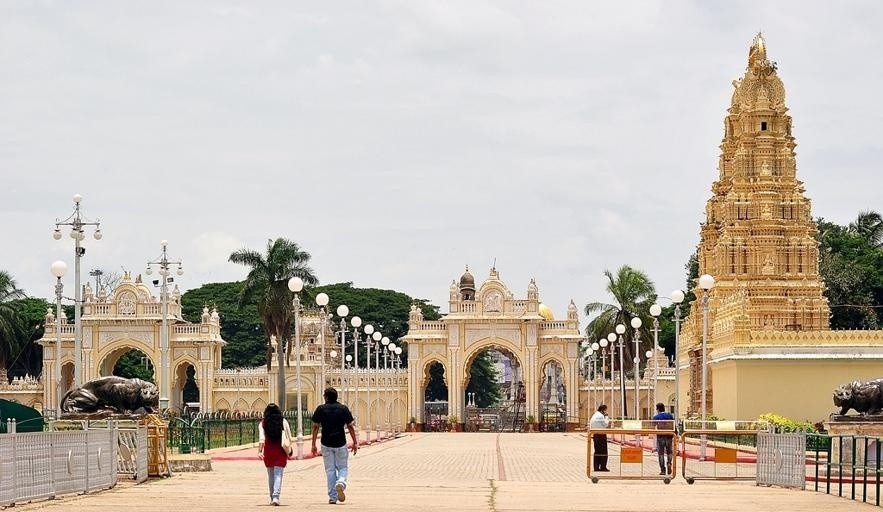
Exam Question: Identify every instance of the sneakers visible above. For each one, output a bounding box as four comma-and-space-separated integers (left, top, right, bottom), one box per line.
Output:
329, 483, 345, 504
271, 498, 279, 505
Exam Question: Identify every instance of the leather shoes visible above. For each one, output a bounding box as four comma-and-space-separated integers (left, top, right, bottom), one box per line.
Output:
594, 468, 609, 471
660, 464, 671, 475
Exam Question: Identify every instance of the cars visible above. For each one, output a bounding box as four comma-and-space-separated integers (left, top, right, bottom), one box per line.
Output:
471, 413, 503, 433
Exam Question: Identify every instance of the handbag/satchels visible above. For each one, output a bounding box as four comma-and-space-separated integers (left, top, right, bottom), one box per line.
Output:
281, 431, 292, 455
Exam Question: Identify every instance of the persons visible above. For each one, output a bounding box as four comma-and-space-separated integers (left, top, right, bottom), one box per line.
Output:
651, 402, 678, 475
589, 405, 611, 471
258, 402, 294, 506
310, 387, 358, 503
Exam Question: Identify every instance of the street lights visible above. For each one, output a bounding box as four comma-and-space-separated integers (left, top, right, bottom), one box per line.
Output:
146, 239, 186, 415
288, 276, 403, 461
577, 273, 715, 445
49, 190, 103, 425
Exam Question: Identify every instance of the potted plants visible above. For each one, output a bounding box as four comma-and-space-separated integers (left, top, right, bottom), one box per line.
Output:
410, 416, 416, 428
449, 414, 458, 429
528, 415, 535, 433
